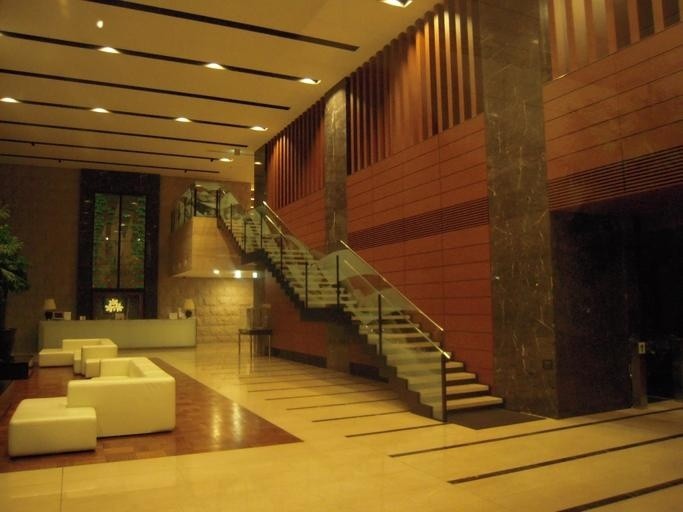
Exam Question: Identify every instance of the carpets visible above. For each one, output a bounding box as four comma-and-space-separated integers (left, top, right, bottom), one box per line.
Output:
0, 356, 304, 475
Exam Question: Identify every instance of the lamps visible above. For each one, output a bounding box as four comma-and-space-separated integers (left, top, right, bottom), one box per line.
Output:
183, 298, 195, 318
43, 298, 57, 320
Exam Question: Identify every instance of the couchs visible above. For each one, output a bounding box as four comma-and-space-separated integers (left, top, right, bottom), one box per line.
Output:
38, 338, 118, 376
8, 356, 176, 457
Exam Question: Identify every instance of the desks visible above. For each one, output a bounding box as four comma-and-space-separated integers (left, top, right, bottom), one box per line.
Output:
238, 328, 273, 362
